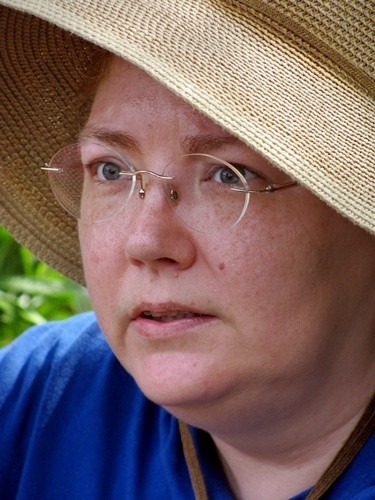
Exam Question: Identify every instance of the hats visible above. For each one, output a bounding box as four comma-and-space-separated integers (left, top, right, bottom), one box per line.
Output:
0, 0, 375, 284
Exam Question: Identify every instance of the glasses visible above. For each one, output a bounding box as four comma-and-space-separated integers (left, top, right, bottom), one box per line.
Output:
40, 139, 297, 236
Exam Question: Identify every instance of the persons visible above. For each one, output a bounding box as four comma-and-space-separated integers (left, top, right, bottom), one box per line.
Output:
0, 0, 375, 500
